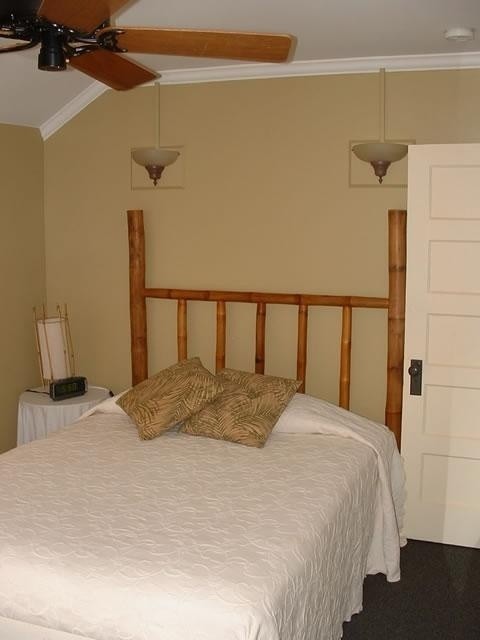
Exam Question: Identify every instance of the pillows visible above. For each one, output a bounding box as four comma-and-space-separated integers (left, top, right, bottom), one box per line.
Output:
114, 355, 306, 450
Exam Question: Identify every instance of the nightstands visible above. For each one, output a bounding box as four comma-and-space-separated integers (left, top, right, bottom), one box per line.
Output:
18, 384, 110, 445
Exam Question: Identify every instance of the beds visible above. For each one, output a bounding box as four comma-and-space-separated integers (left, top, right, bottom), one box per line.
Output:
0, 207, 409, 640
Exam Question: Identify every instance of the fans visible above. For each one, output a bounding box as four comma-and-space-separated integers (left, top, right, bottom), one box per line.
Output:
1, 0, 300, 94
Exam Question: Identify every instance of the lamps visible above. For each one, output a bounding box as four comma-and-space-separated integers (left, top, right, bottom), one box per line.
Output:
129, 81, 181, 188
31, 302, 76, 390
351, 66, 409, 186
